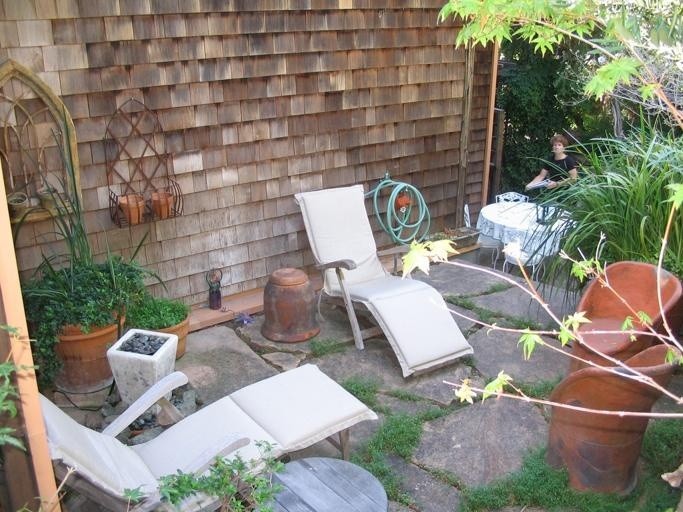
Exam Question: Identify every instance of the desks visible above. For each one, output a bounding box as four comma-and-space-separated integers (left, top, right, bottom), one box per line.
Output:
478, 200, 573, 280
253, 453, 392, 511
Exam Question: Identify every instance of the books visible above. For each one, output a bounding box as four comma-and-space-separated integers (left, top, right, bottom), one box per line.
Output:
526, 179, 550, 190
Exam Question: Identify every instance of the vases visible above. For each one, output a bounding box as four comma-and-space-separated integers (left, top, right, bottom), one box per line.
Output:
121, 190, 176, 223
105, 329, 178, 415
7, 186, 58, 215
259, 266, 320, 341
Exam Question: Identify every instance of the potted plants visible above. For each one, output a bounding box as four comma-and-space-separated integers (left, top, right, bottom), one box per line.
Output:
12, 103, 173, 393
128, 293, 192, 360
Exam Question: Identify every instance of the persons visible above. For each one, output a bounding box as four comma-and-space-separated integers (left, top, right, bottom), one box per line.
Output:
525, 137, 577, 203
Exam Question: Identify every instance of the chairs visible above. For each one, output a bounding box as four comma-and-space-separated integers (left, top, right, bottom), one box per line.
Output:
291, 184, 479, 379
24, 361, 378, 511
549, 258, 682, 498
495, 191, 530, 203
501, 229, 546, 281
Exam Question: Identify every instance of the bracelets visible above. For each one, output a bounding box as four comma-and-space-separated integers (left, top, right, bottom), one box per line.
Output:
555, 182, 560, 188
